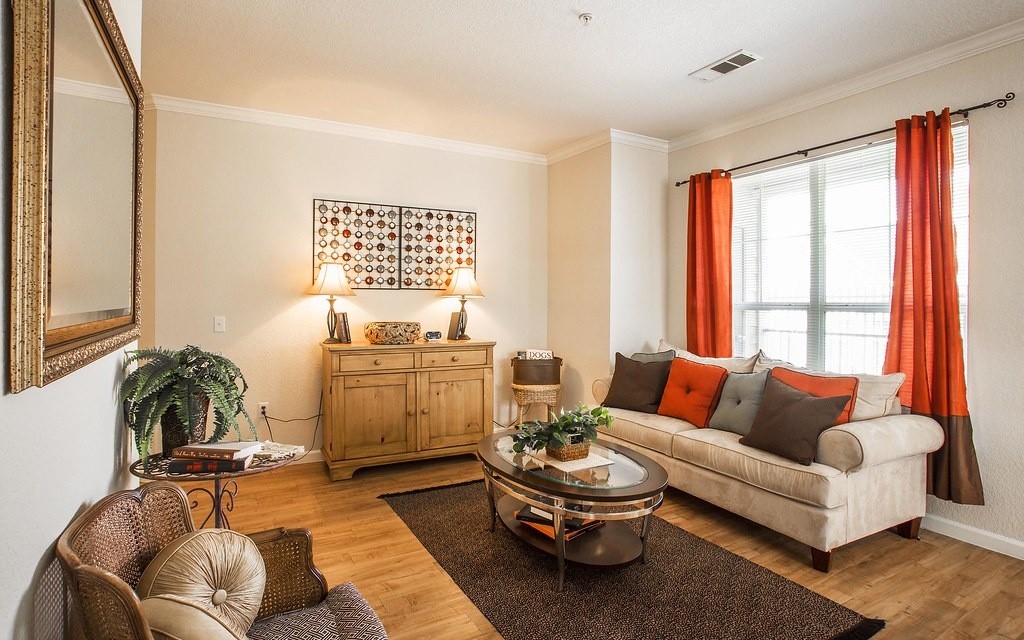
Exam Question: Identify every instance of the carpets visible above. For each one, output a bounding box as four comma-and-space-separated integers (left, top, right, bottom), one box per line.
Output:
377, 464, 887, 640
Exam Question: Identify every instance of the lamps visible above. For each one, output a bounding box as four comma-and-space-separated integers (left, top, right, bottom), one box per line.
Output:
308, 263, 360, 346
441, 266, 485, 340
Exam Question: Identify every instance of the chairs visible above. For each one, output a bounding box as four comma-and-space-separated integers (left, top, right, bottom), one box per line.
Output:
54, 478, 388, 640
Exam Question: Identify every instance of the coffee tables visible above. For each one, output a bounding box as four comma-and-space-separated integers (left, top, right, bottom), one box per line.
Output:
477, 427, 668, 592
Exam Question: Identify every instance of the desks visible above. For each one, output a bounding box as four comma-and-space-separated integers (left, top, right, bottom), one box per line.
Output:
130, 452, 296, 531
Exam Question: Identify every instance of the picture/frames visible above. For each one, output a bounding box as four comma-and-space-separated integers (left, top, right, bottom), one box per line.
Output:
10, 0, 146, 393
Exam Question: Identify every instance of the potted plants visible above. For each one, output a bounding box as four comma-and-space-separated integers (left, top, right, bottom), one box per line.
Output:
121, 343, 260, 475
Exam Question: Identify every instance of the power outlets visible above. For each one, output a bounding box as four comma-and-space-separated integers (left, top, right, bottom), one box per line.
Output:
259, 402, 268, 417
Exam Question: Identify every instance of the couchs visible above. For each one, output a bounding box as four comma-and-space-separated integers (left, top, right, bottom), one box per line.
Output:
592, 377, 945, 571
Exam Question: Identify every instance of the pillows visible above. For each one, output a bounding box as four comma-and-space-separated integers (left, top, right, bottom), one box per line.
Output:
137, 527, 265, 640
600, 338, 905, 466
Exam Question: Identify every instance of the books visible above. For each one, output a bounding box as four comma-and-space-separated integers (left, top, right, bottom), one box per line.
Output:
171, 440, 262, 461
513, 494, 606, 541
167, 454, 254, 472
335, 312, 352, 344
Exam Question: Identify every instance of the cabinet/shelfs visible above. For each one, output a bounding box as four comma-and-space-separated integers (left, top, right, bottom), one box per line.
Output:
318, 340, 497, 482
